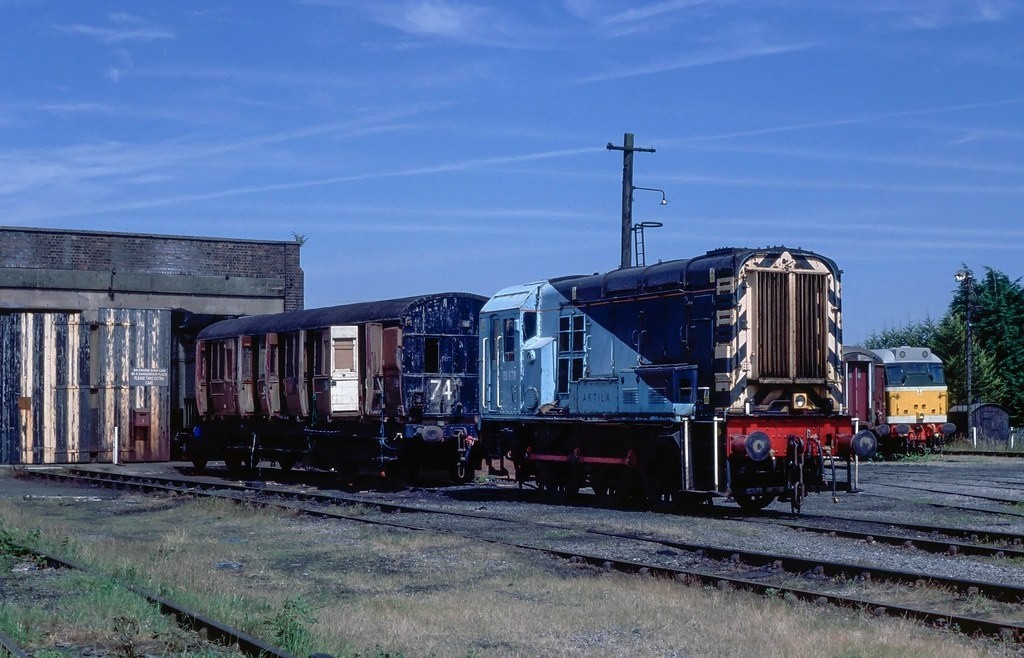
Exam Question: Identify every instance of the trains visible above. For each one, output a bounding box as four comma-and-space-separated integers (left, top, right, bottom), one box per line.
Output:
843, 346, 955, 455
175, 245, 852, 513
